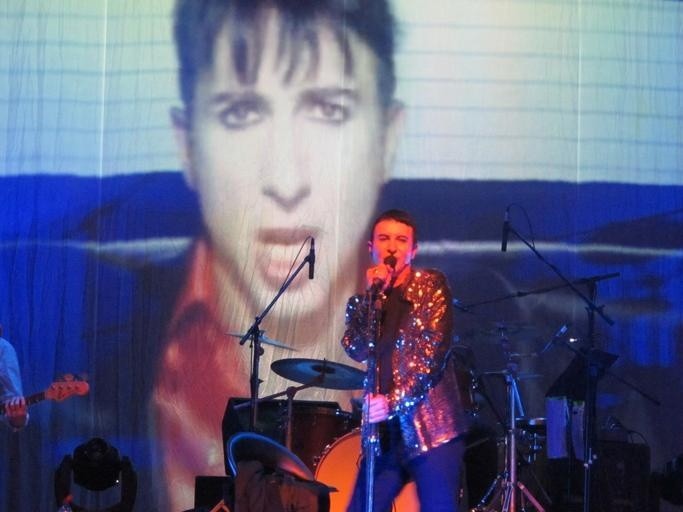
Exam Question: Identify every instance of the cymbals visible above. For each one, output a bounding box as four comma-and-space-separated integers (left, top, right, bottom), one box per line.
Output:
272, 358, 366, 390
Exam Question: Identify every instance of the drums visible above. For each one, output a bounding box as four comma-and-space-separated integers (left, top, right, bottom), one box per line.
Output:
454, 364, 479, 415
301, 405, 349, 463
316, 426, 467, 511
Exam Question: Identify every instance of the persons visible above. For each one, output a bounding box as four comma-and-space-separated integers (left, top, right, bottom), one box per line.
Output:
143, 1, 406, 512
341, 209, 472, 511
1, 338, 29, 511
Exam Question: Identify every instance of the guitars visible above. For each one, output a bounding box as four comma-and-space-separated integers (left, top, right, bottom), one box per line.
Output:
1, 376, 89, 414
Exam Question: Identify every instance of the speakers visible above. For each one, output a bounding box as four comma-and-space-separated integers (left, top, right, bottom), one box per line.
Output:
584, 442, 651, 512
221, 397, 351, 476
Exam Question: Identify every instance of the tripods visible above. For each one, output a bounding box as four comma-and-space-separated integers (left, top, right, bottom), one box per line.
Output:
470, 335, 553, 512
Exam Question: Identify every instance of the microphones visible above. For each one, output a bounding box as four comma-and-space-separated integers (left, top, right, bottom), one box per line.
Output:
543, 320, 569, 352
502, 208, 509, 251
452, 298, 469, 311
373, 256, 396, 289
309, 239, 315, 279
